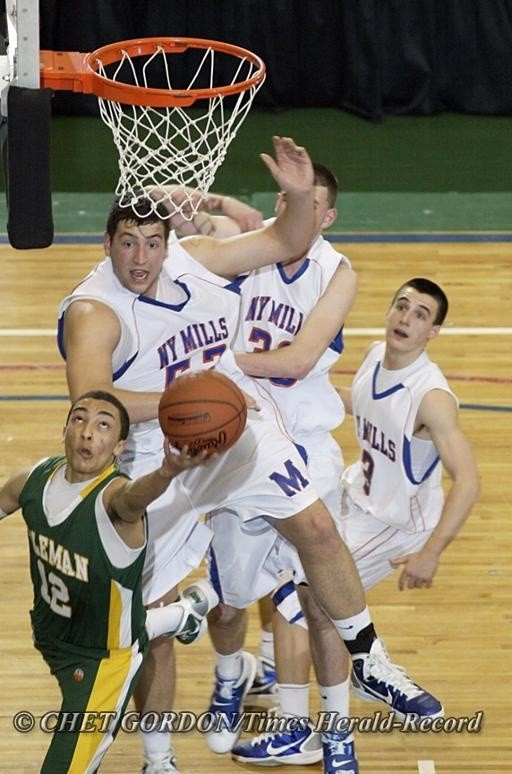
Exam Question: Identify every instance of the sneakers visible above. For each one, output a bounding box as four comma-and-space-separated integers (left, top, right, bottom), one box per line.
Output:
141, 638, 445, 773
166, 580, 220, 645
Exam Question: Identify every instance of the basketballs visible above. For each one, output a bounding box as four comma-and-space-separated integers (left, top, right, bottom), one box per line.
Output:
159, 370, 247, 459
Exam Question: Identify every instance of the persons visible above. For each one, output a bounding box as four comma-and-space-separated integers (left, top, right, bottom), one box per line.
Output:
0, 137, 479, 772
